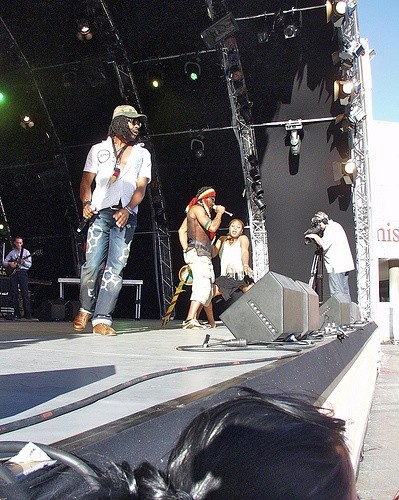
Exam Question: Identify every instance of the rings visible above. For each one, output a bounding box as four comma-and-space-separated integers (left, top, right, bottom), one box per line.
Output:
119, 212, 123, 215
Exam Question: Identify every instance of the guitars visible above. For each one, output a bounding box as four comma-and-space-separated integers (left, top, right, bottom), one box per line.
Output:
5, 249, 43, 277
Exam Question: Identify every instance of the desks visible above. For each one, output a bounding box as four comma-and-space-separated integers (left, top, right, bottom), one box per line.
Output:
57, 277, 144, 320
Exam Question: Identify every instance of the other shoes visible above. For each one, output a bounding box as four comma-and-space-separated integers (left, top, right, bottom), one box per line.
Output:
182, 319, 206, 330
25, 313, 30, 318
15, 312, 21, 318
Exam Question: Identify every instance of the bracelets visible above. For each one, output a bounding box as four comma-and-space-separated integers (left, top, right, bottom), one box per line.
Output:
244, 264, 249, 267
124, 206, 136, 216
82, 201, 91, 208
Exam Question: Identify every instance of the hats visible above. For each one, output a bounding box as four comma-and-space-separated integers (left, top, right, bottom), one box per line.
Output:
113, 105, 147, 122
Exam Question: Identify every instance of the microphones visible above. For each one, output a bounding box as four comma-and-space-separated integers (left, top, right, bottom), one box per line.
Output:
76, 204, 96, 232
212, 205, 233, 217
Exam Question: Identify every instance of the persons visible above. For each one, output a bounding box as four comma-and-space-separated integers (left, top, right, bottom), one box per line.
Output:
304, 211, 356, 331
76, 385, 361, 500
73, 105, 155, 333
2, 236, 31, 320
178, 185, 224, 329
211, 219, 255, 304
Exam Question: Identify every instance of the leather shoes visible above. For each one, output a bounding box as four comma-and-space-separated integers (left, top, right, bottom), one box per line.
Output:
73, 311, 91, 331
93, 323, 117, 336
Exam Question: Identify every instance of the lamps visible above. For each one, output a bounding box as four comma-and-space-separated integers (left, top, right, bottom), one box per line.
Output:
248, 155, 266, 210
339, 80, 353, 99
330, 1, 347, 23
75, 15, 92, 34
147, 64, 164, 89
285, 123, 303, 155
231, 63, 253, 125
281, 12, 299, 39
257, 16, 275, 43
188, 135, 207, 160
150, 180, 168, 228
38, 172, 48, 178
184, 56, 201, 82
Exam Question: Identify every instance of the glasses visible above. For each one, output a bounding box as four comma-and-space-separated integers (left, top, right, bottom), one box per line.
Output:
126, 117, 141, 126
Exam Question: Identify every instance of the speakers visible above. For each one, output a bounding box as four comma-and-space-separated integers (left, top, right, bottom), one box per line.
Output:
0, 275, 13, 307
318, 293, 351, 326
350, 302, 362, 325
296, 280, 321, 333
218, 270, 309, 346
